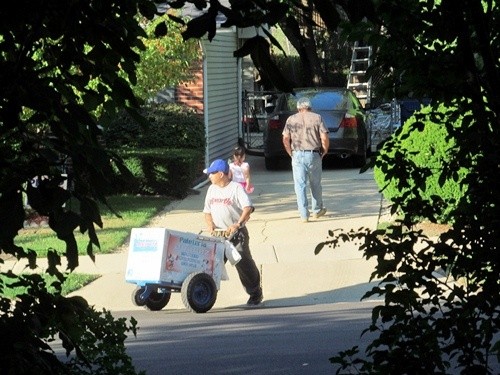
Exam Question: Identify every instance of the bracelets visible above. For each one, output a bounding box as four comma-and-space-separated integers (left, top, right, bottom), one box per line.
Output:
238, 221, 242, 226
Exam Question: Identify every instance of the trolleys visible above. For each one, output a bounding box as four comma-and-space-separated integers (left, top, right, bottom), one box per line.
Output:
126, 228, 234, 313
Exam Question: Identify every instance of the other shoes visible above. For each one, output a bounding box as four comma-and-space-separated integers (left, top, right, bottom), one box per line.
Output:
312, 208, 327, 219
247, 294, 263, 305
301, 218, 308, 223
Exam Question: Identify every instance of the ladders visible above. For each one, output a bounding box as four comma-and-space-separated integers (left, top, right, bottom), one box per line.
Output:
347, 40, 373, 111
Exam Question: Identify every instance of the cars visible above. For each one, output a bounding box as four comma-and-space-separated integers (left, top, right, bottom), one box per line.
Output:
264, 87, 374, 169
245, 96, 272, 130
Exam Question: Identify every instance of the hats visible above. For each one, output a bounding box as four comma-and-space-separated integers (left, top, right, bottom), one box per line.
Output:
203, 159, 229, 174
297, 97, 312, 108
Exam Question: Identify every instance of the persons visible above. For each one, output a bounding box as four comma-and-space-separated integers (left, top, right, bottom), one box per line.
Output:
203, 159, 264, 306
281, 96, 330, 223
229, 146, 254, 206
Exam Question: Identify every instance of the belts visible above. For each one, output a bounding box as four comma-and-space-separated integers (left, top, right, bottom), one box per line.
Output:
292, 150, 319, 152
